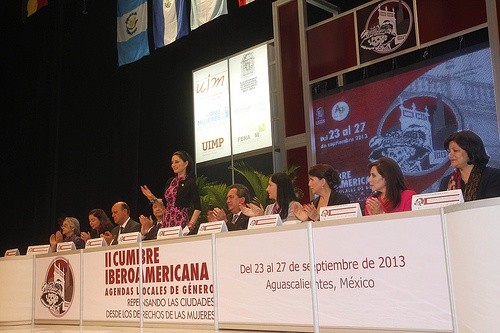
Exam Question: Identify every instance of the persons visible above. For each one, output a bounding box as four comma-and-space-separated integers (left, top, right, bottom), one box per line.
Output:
364, 157, 415, 216
439, 130, 500, 202
48, 198, 164, 252
142, 150, 202, 237
207, 165, 350, 232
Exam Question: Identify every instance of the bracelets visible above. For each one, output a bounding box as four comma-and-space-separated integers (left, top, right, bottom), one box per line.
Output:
151, 197, 157, 205
382, 212, 386, 214
188, 223, 196, 231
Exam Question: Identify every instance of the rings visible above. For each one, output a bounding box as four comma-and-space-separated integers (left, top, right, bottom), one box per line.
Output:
376, 203, 378, 206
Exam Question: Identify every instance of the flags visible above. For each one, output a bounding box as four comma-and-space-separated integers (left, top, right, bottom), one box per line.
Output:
117, 0, 253, 67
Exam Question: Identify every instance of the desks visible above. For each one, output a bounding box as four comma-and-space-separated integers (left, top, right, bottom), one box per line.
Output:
0, 197, 500, 333
